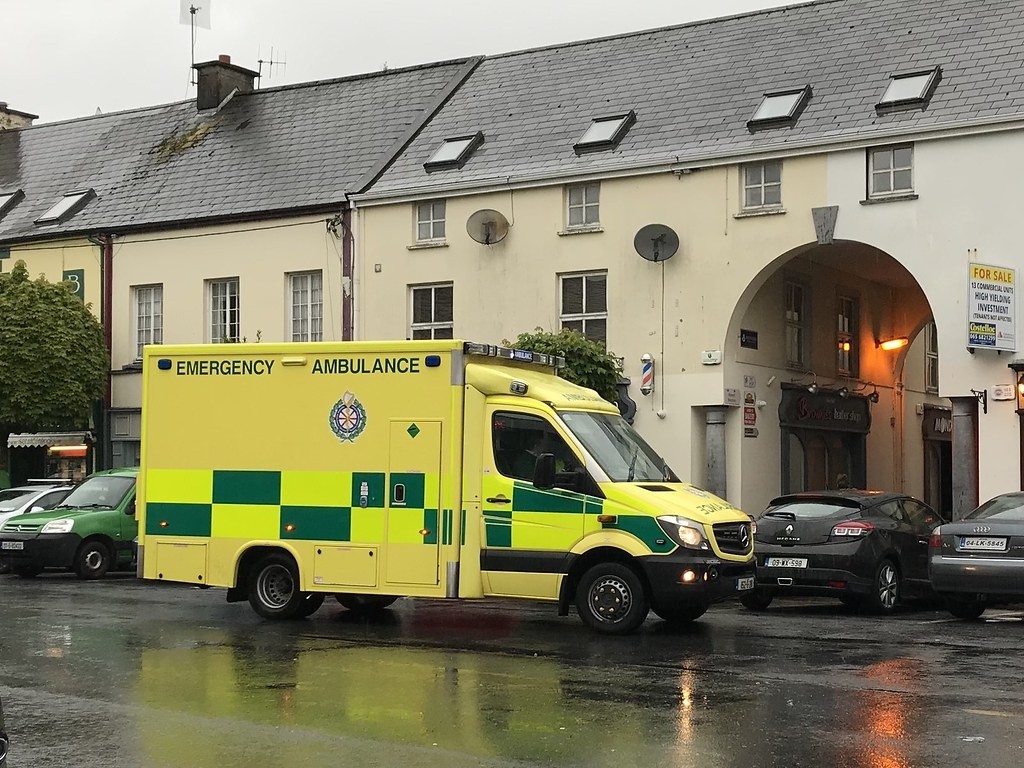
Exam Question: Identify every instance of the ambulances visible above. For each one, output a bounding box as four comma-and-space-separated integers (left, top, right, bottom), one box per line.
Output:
134, 339, 757, 634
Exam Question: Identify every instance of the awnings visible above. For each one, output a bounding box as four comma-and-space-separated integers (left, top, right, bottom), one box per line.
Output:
7, 429, 98, 449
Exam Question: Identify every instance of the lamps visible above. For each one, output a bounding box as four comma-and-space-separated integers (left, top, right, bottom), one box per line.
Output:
874, 336, 909, 351
852, 381, 879, 403
822, 373, 849, 399
791, 371, 819, 396
1017, 373, 1024, 397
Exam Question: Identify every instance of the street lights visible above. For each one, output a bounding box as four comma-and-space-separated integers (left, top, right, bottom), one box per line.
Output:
1006, 360, 1024, 491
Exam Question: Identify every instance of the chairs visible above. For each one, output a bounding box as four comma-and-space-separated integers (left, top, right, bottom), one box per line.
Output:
496, 427, 525, 476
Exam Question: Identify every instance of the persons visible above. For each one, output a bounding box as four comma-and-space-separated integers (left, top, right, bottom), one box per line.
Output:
510, 428, 550, 482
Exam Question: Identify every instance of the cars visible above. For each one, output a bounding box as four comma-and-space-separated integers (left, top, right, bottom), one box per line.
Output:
1, 467, 138, 578
928, 490, 1023, 618
743, 489, 946, 615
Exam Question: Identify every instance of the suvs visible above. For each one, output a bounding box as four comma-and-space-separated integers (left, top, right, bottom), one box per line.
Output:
1, 478, 76, 530
